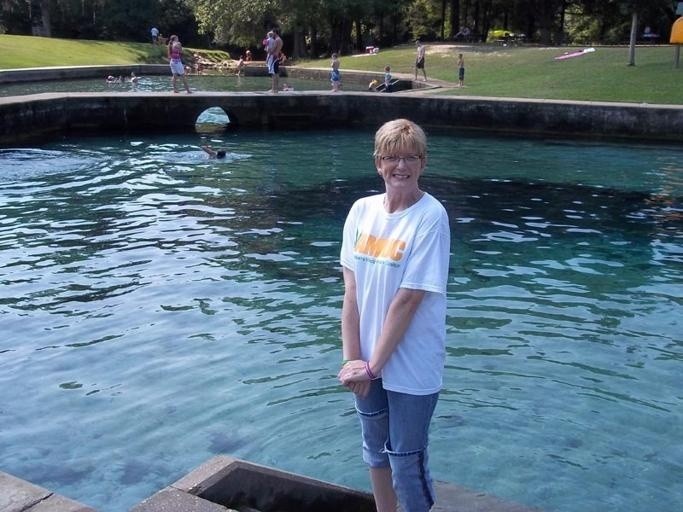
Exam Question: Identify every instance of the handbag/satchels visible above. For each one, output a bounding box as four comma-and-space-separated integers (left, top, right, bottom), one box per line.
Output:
365, 361, 376, 380
340, 360, 351, 365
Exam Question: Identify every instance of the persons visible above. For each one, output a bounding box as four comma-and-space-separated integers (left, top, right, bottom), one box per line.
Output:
383, 65, 392, 93
235, 50, 252, 76
415, 40, 428, 82
150, 26, 159, 46
106, 71, 139, 84
330, 53, 340, 93
337, 118, 452, 512
168, 34, 192, 94
200, 144, 227, 160
183, 62, 204, 75
263, 28, 292, 94
457, 54, 465, 87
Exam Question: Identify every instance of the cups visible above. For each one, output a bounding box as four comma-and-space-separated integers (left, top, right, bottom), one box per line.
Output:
374, 154, 424, 163
